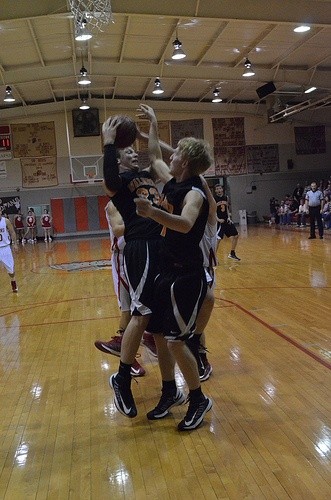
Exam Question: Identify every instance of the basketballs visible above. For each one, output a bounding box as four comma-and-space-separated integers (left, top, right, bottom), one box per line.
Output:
108, 114, 139, 149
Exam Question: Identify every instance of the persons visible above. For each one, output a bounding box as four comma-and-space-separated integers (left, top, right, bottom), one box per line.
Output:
268, 180, 331, 229
0, 206, 18, 292
94, 104, 216, 431
213, 185, 241, 261
14, 208, 53, 243
305, 181, 324, 239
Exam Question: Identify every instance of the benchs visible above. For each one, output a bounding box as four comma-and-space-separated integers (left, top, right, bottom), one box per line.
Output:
290, 210, 310, 223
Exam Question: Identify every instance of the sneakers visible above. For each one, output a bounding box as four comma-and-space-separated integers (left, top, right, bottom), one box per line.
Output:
178, 395, 212, 431
147, 388, 185, 420
11, 281, 18, 292
228, 253, 241, 261
199, 359, 212, 382
109, 372, 137, 417
95, 335, 123, 357
130, 359, 146, 376
139, 333, 158, 358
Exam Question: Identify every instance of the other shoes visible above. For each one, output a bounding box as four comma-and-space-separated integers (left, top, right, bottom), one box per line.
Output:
16, 238, 52, 242
320, 236, 323, 239
308, 236, 316, 239
297, 225, 306, 228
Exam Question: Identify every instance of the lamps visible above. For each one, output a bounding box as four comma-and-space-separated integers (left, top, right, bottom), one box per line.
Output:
171, 29, 186, 59
77, 50, 91, 85
3, 86, 16, 102
75, 18, 92, 40
79, 97, 90, 109
152, 79, 164, 94
242, 58, 255, 77
212, 89, 223, 103
